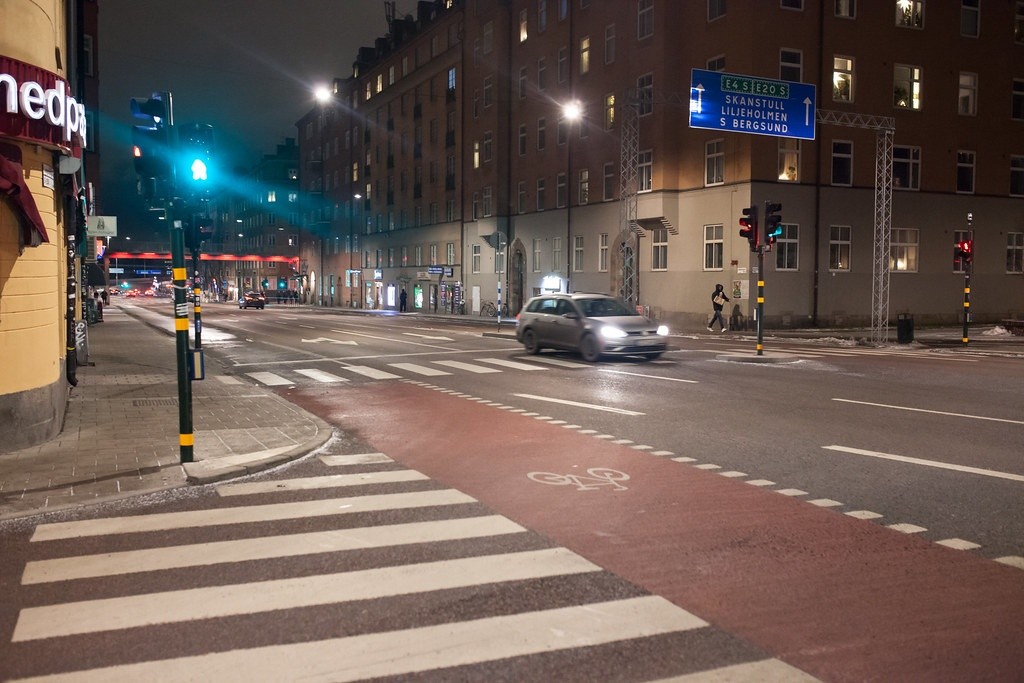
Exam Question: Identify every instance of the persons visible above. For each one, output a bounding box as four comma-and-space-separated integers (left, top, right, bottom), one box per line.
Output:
276, 288, 298, 304
706, 284, 729, 332
94, 291, 98, 298
400, 289, 407, 312
100, 290, 108, 305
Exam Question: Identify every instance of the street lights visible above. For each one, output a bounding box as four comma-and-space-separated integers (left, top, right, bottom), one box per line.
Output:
565, 102, 581, 293
318, 89, 328, 306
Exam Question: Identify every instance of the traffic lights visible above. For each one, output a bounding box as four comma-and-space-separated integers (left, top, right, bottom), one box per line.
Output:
959, 240, 970, 257
131, 97, 168, 176
740, 208, 757, 242
178, 124, 215, 198
766, 202, 782, 237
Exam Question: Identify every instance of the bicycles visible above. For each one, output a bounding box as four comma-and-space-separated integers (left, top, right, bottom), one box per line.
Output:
480, 299, 507, 318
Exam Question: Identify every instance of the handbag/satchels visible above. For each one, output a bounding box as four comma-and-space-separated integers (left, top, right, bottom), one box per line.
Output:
713, 292, 725, 305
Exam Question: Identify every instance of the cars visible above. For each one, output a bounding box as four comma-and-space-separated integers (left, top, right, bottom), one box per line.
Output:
239, 292, 264, 309
516, 293, 667, 362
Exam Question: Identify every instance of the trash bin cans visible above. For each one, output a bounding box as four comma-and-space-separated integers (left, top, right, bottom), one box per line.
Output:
897, 312, 915, 344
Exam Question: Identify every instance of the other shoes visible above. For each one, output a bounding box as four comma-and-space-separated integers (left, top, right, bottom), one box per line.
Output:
707, 327, 714, 332
721, 328, 727, 332
